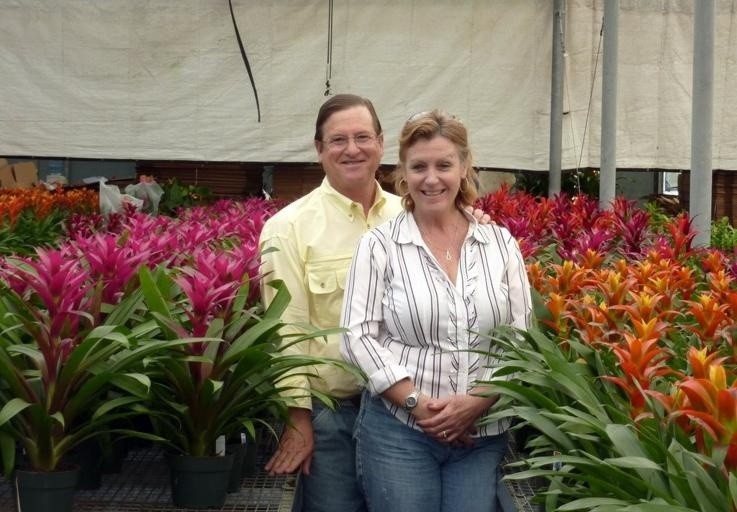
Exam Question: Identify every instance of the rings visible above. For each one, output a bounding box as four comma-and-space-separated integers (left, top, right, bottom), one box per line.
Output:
443, 430, 448, 439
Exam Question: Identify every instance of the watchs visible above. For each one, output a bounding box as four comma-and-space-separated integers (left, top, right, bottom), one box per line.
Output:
401, 390, 421, 414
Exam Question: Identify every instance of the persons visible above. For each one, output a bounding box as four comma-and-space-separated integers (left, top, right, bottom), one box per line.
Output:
340, 112, 533, 512
258, 94, 498, 511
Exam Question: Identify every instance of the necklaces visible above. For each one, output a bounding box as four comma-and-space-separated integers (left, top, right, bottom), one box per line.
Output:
417, 216, 461, 261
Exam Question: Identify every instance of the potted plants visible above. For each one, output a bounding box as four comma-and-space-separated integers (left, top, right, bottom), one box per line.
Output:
0, 166, 306, 510
